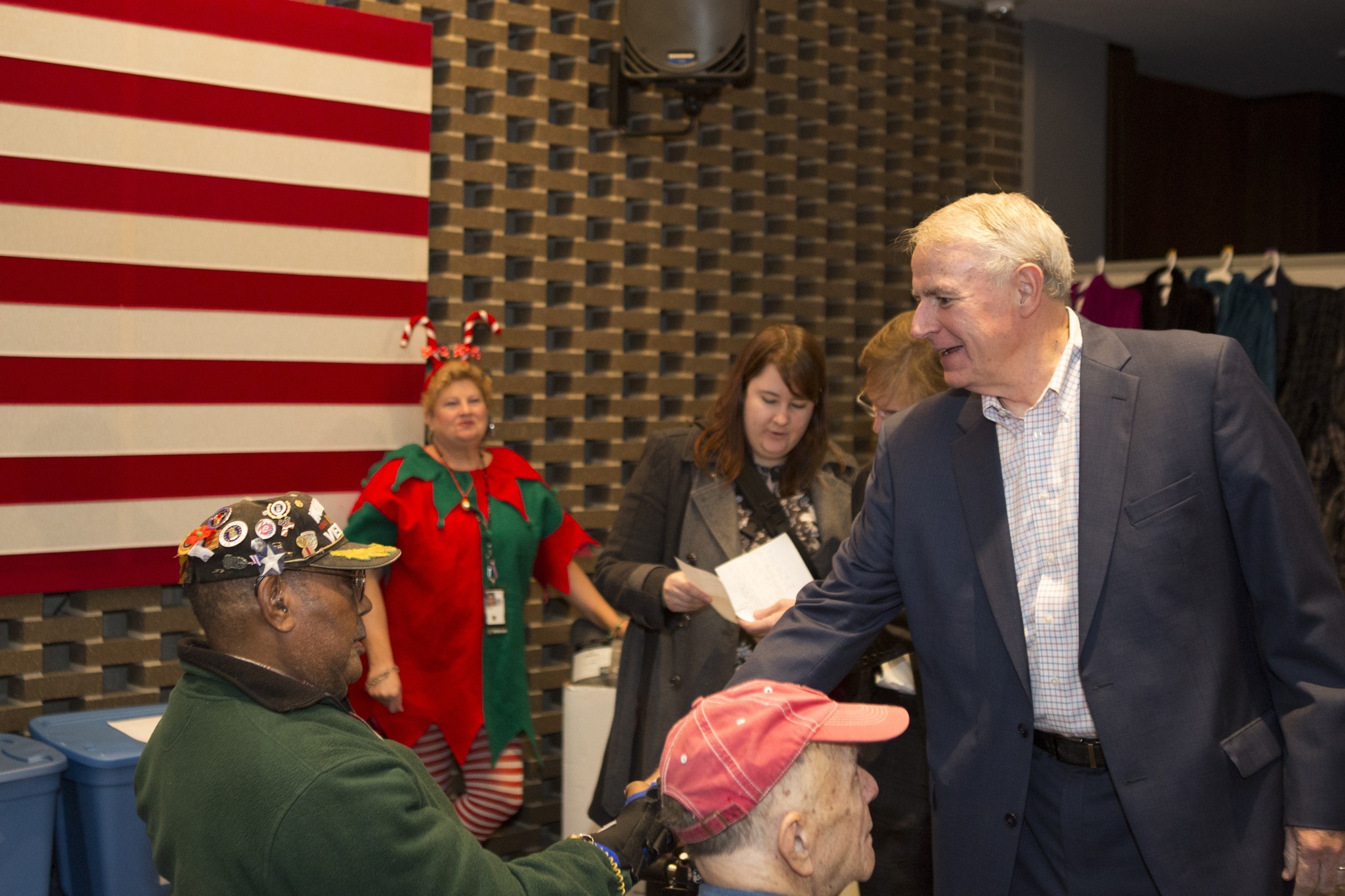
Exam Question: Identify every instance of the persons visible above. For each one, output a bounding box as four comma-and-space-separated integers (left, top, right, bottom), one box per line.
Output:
592, 322, 860, 828
854, 310, 950, 446
656, 678, 910, 896
342, 360, 630, 844
136, 492, 672, 896
652, 192, 1345, 896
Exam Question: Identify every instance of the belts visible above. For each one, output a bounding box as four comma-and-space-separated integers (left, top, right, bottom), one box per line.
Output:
1030, 732, 1109, 768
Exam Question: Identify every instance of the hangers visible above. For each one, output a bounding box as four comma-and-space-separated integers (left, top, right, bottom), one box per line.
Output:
1077, 246, 1286, 293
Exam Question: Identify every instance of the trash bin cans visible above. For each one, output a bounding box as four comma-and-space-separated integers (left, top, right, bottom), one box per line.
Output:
0, 731, 67, 896
27, 702, 172, 896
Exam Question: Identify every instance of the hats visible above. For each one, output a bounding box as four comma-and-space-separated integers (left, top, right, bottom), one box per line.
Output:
173, 491, 402, 586
656, 676, 909, 849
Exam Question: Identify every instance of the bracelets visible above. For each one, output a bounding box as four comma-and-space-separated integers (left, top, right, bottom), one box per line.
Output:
365, 666, 401, 691
607, 618, 626, 641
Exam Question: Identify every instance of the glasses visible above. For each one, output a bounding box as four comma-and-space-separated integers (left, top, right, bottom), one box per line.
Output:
854, 385, 900, 420
280, 565, 366, 604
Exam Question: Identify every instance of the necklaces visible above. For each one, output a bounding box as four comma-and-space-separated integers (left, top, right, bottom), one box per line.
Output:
431, 442, 483, 511
